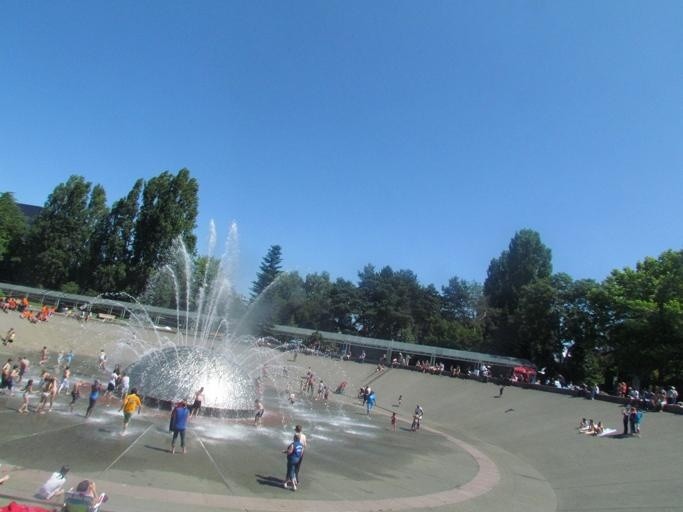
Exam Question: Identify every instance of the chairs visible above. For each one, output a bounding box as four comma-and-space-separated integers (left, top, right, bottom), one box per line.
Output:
63, 491, 94, 511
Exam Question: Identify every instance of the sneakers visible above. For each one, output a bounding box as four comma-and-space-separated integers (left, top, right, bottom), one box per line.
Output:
280, 483, 297, 491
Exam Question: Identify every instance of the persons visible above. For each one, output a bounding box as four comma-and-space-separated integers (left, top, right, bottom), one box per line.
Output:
169, 400, 189, 454
0, 474, 10, 485
253, 336, 491, 491
66, 479, 108, 512
38, 465, 70, 501
0, 346, 143, 435
0, 297, 88, 325
500, 373, 678, 436
190, 387, 204, 420
3, 328, 16, 345
